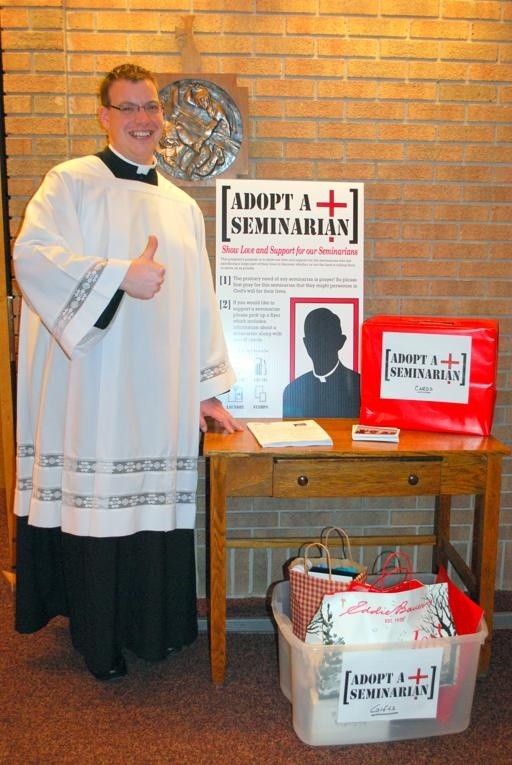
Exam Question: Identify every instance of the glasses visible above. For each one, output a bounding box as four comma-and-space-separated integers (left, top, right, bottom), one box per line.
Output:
105, 102, 164, 116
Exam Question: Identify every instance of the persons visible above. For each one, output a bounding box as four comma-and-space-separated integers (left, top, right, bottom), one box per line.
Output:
12, 63, 244, 680
283, 309, 361, 417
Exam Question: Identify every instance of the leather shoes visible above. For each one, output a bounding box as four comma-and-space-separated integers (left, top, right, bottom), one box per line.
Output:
85, 650, 126, 680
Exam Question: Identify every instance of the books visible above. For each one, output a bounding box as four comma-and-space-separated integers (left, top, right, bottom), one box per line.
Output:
247, 419, 332, 448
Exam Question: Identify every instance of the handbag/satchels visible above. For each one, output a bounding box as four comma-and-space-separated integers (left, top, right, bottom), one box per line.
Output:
305, 551, 484, 643
288, 526, 367, 641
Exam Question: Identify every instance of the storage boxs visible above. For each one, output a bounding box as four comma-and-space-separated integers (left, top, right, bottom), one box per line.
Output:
271, 573, 489, 747
361, 313, 500, 438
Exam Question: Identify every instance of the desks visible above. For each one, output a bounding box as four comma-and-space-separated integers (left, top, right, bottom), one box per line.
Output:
203, 418, 512, 687
